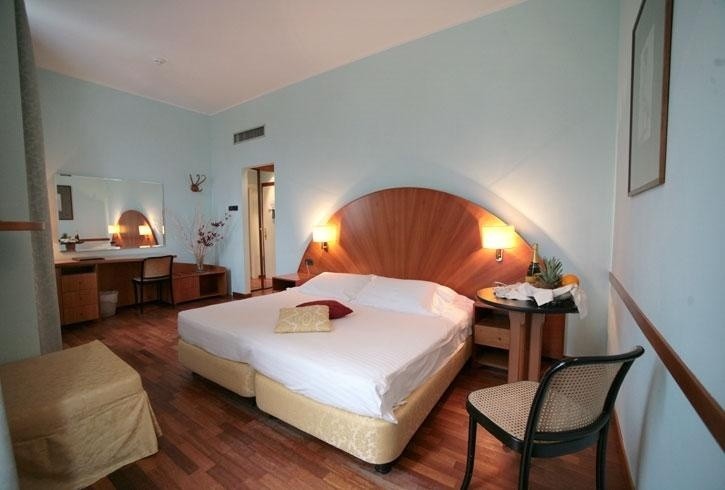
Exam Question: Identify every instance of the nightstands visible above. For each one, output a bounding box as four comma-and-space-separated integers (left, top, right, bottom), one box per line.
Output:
471, 299, 510, 371
270, 272, 305, 292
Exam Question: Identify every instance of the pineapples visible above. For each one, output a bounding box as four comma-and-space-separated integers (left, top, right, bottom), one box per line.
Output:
533, 255, 565, 289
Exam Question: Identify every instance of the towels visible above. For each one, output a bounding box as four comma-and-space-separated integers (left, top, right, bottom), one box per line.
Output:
498, 279, 588, 319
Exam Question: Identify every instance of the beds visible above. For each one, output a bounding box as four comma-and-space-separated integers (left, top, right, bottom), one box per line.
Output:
176, 186, 567, 476
61, 210, 160, 251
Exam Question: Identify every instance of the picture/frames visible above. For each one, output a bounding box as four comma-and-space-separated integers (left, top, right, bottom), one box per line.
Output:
627, 0, 672, 197
56, 184, 73, 221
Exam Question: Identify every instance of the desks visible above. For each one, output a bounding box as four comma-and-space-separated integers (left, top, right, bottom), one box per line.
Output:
58, 234, 78, 250
476, 284, 583, 385
54, 251, 178, 329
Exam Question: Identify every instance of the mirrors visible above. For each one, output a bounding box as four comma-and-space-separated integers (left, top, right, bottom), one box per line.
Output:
52, 171, 168, 249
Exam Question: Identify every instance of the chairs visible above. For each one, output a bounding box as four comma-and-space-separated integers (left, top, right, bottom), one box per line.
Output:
463, 347, 643, 488
132, 254, 175, 316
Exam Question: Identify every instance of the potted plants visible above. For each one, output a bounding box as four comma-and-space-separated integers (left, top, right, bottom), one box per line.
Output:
534, 259, 563, 288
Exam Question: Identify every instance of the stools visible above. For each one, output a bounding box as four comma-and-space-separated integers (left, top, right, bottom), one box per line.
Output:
2, 338, 163, 490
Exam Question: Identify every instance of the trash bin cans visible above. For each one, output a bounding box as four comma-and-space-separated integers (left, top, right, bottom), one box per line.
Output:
99, 291, 119, 319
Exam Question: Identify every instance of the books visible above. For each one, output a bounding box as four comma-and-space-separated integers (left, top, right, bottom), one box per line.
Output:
71, 255, 104, 262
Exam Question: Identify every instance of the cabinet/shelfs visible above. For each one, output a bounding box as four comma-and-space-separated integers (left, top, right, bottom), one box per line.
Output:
171, 266, 227, 306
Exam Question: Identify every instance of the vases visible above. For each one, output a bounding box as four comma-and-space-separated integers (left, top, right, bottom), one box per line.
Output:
194, 251, 207, 271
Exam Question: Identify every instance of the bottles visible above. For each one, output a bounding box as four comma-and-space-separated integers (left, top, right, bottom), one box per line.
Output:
525, 241, 541, 286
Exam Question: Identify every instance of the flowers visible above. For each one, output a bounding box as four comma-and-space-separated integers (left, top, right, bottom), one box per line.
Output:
178, 212, 235, 251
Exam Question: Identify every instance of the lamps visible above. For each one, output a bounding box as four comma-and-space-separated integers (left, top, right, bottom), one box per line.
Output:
481, 224, 517, 261
309, 222, 341, 253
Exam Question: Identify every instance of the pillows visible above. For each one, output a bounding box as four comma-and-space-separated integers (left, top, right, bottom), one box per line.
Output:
296, 300, 352, 320
274, 303, 331, 334
285, 268, 376, 305
351, 271, 456, 318
